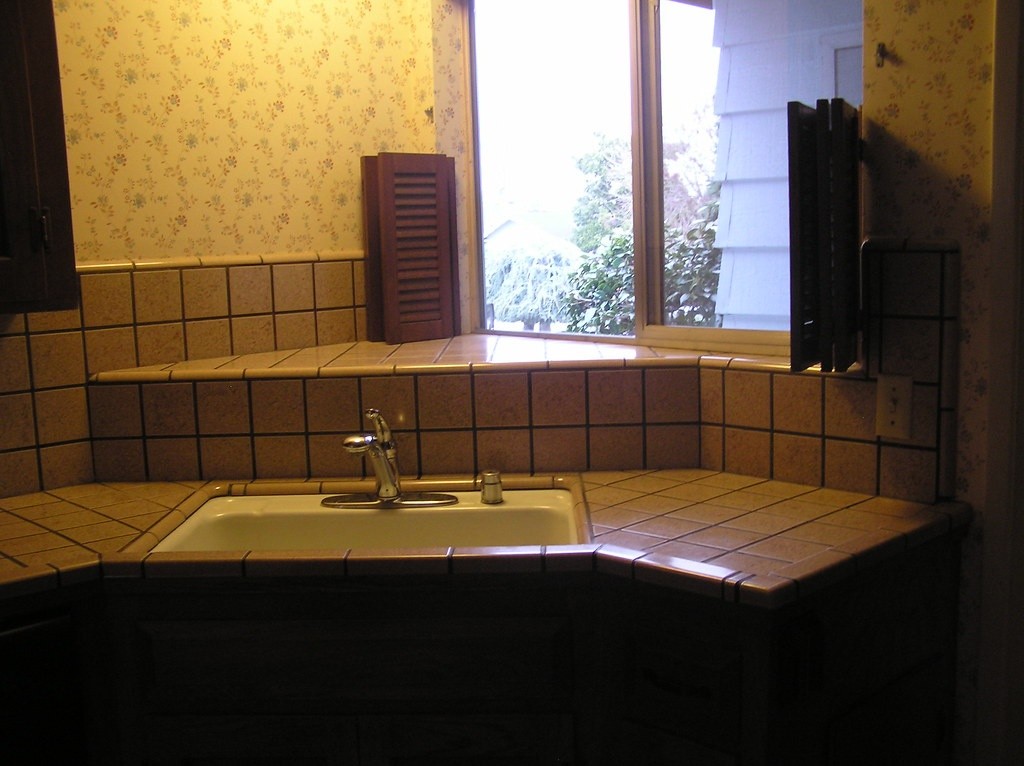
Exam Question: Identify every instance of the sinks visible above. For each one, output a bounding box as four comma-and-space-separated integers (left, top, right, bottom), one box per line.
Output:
148, 504, 579, 552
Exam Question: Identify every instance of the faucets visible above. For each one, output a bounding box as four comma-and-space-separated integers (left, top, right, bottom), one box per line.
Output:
343, 408, 402, 502
